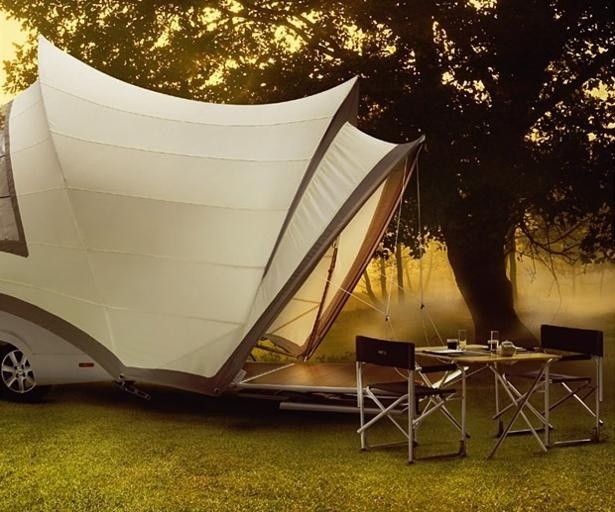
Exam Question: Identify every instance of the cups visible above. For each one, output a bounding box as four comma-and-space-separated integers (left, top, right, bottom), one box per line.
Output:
447, 330, 466, 350
487, 330, 499, 351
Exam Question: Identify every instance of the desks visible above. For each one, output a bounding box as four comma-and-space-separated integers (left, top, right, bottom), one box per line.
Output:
413, 344, 562, 459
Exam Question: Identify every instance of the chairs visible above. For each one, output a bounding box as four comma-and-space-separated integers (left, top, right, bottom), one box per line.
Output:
355, 335, 470, 463
495, 324, 604, 448
